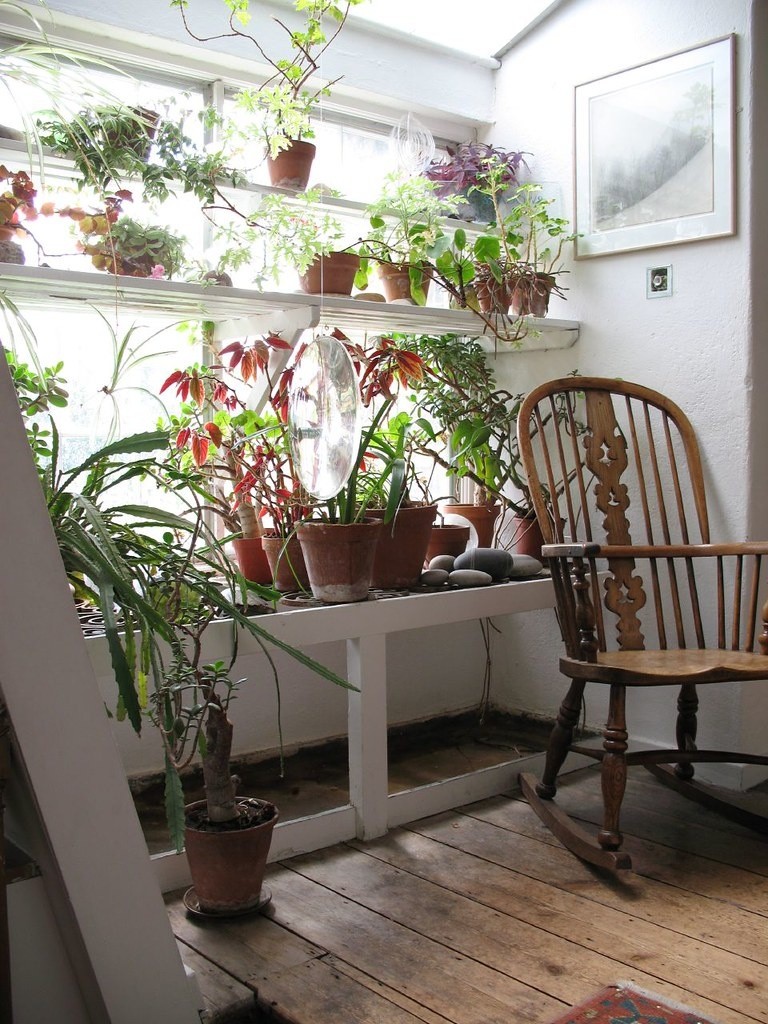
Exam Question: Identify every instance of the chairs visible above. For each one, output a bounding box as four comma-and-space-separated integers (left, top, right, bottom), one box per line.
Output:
513, 377, 768, 901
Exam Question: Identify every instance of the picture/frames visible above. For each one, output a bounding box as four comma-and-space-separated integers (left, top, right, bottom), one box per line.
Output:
572, 32, 738, 261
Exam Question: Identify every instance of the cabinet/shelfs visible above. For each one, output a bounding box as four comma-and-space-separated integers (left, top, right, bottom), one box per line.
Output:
0, 138, 607, 895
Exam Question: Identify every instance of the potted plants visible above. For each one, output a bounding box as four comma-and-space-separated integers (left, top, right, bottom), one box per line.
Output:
0, 0, 584, 605
103, 575, 281, 912
